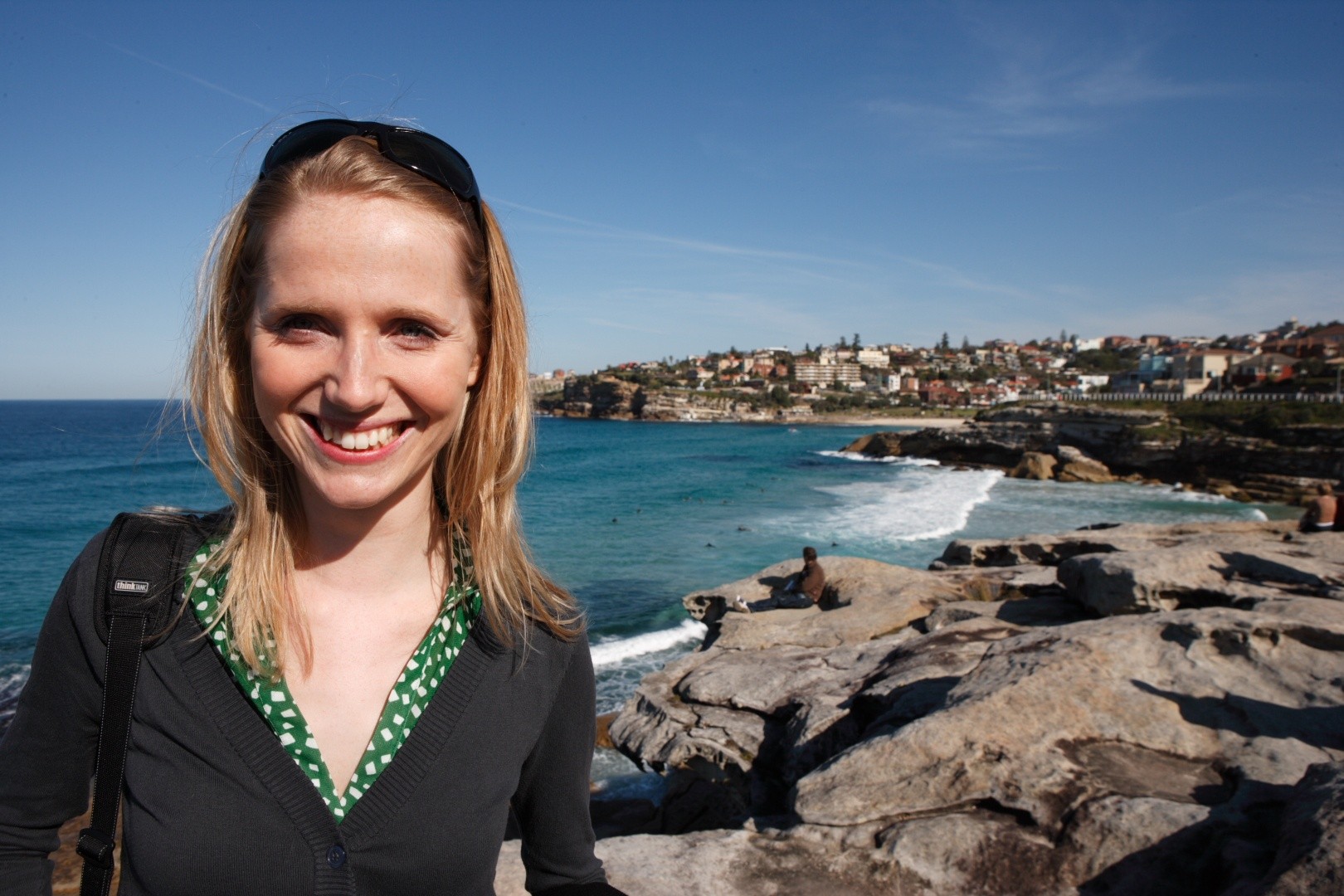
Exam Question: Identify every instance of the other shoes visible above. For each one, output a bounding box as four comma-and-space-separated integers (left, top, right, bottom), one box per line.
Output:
734, 596, 751, 613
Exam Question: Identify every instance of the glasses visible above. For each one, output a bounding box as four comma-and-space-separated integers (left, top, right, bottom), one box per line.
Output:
257, 120, 482, 230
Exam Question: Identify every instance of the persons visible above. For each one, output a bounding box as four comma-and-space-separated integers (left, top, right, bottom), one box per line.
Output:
0, 119, 628, 896
1297, 482, 1344, 532
732, 547, 825, 613
